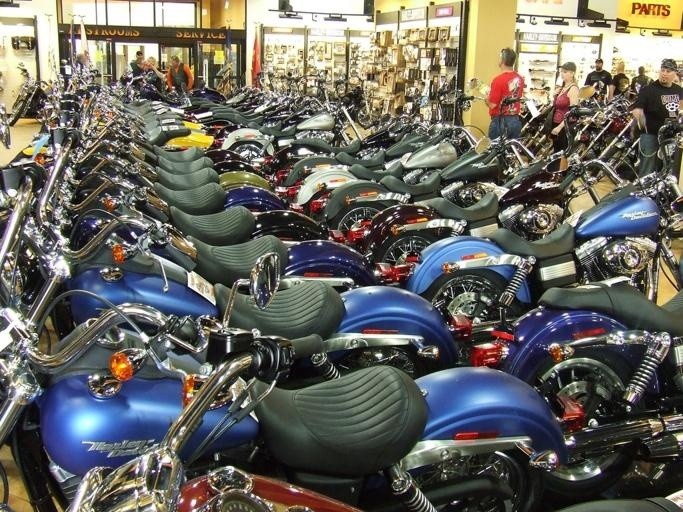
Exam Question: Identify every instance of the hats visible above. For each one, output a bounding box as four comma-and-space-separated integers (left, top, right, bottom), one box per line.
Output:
558, 62, 576, 71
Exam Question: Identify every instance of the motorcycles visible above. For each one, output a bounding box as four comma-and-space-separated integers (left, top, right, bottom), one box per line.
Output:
0, 58, 682, 505
0, 162, 566, 510
60, 332, 364, 511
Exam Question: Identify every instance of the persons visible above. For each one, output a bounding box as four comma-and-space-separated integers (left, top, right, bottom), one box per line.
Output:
484, 47, 525, 140
550, 60, 580, 171
583, 57, 683, 196
128, 51, 194, 92
